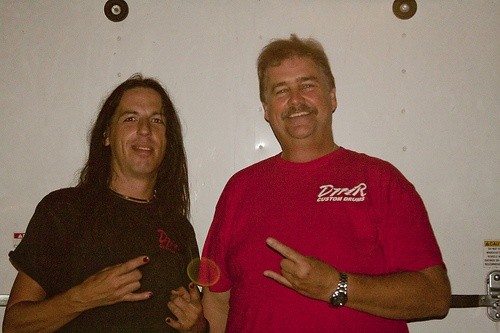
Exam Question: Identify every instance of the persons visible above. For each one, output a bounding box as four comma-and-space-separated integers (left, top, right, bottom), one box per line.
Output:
2, 73, 207, 333
195, 33, 452, 333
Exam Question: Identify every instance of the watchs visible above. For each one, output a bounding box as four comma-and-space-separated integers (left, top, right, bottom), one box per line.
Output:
329, 269, 350, 309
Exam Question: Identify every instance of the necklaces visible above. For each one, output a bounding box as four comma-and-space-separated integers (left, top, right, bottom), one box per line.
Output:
107, 188, 159, 203
